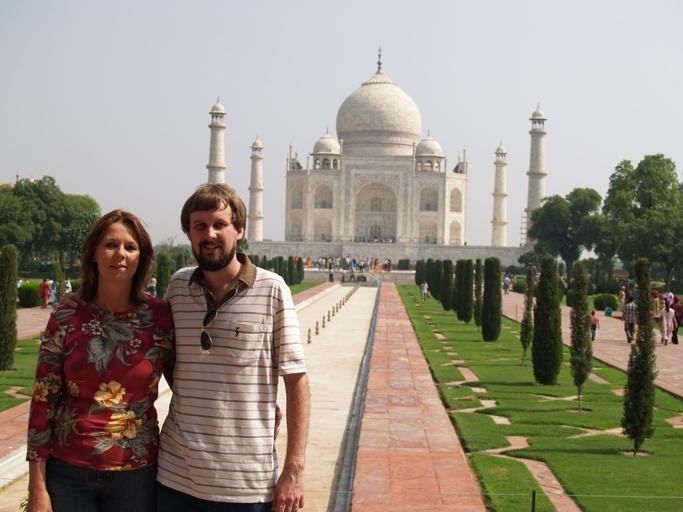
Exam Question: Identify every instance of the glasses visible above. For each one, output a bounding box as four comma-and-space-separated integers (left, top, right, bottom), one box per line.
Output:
200, 309, 217, 351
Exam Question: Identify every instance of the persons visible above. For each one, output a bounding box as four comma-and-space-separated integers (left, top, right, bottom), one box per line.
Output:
26, 210, 282, 512
61, 182, 311, 512
